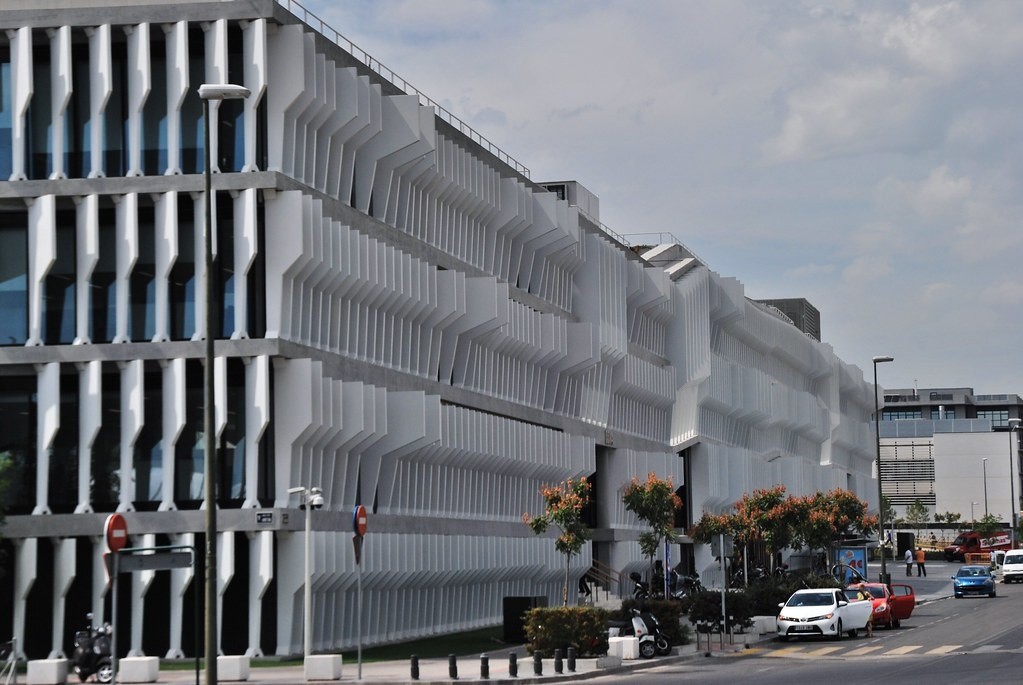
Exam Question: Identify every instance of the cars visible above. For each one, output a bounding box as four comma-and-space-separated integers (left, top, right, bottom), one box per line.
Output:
949, 566, 998, 597
775, 587, 873, 640
849, 581, 917, 631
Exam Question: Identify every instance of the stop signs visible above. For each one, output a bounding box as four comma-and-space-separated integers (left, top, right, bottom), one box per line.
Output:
106, 518, 128, 550
357, 507, 367, 535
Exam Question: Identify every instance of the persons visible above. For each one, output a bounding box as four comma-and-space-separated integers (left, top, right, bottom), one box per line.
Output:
904, 545, 914, 577
915, 547, 927, 577
885, 531, 893, 546
990, 550, 995, 569
928, 532, 938, 550
857, 584, 875, 637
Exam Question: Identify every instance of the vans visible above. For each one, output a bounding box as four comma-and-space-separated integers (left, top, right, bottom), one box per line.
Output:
994, 550, 1023, 584
943, 528, 1012, 562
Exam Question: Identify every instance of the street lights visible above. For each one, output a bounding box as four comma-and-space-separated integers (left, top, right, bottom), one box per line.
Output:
1008, 418, 1022, 548
198, 83, 251, 685
288, 487, 314, 680
983, 458, 988, 522
874, 354, 894, 579
972, 502, 979, 531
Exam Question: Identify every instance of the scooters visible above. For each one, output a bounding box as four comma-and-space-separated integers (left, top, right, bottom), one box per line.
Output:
630, 572, 670, 599
72, 614, 118, 683
600, 582, 673, 658
731, 563, 790, 589
